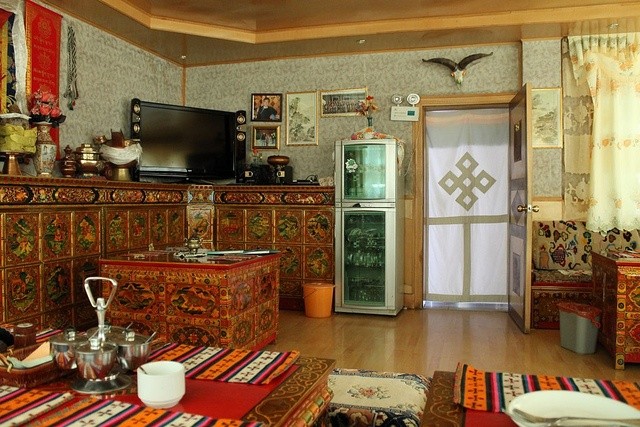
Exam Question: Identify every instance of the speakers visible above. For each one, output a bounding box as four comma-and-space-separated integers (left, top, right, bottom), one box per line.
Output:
233, 110, 249, 186
128, 98, 151, 183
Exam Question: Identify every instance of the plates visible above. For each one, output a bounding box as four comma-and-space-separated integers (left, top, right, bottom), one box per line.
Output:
508, 389, 640, 427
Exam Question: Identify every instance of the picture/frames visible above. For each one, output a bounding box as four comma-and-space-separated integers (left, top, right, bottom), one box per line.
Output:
250, 124, 281, 151
250, 93, 283, 122
285, 90, 319, 147
529, 87, 563, 149
320, 85, 369, 118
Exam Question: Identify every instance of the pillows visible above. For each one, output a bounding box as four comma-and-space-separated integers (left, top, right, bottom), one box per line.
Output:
585, 228, 605, 258
586, 227, 640, 263
534, 219, 594, 270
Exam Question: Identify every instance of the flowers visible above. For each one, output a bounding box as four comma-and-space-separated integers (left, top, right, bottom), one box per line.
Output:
354, 96, 378, 116
27, 88, 62, 121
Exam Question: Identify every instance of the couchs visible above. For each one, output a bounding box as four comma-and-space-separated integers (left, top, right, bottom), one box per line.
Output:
531, 261, 594, 330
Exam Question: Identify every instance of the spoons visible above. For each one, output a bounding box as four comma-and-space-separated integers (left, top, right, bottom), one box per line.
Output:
513, 406, 640, 427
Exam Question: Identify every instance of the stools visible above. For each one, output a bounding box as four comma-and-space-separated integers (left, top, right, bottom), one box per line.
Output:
321, 365, 431, 426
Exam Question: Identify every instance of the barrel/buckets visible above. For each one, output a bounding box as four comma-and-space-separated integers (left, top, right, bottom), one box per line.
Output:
301, 281, 336, 318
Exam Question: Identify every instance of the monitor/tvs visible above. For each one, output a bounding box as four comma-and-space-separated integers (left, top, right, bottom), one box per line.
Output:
140, 101, 236, 185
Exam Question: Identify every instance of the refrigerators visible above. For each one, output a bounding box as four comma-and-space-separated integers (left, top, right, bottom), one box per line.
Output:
334, 139, 405, 317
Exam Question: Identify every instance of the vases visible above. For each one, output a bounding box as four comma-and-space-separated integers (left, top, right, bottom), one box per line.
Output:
368, 118, 373, 128
33, 122, 57, 176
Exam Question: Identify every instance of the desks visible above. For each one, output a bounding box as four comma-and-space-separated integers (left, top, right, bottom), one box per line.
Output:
98, 248, 284, 349
590, 249, 640, 371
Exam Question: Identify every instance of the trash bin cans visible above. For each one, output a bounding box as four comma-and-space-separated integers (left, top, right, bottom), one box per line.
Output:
556, 302, 602, 354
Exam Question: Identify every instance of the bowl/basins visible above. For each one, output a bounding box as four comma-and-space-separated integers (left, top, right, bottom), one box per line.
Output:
87, 325, 126, 343
109, 332, 151, 370
50, 328, 89, 370
73, 337, 117, 382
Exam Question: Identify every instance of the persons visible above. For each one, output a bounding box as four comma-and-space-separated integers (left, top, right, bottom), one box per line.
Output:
257, 97, 277, 119
264, 133, 269, 146
324, 99, 356, 113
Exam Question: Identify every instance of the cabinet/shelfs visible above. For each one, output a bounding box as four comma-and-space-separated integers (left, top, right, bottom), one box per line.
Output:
0, 173, 106, 341
215, 184, 335, 311
105, 180, 188, 256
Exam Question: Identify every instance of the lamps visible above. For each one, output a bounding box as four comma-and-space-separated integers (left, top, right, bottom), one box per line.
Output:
391, 94, 403, 105
407, 94, 420, 106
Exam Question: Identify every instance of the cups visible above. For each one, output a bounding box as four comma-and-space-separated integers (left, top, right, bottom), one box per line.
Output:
136, 361, 186, 408
